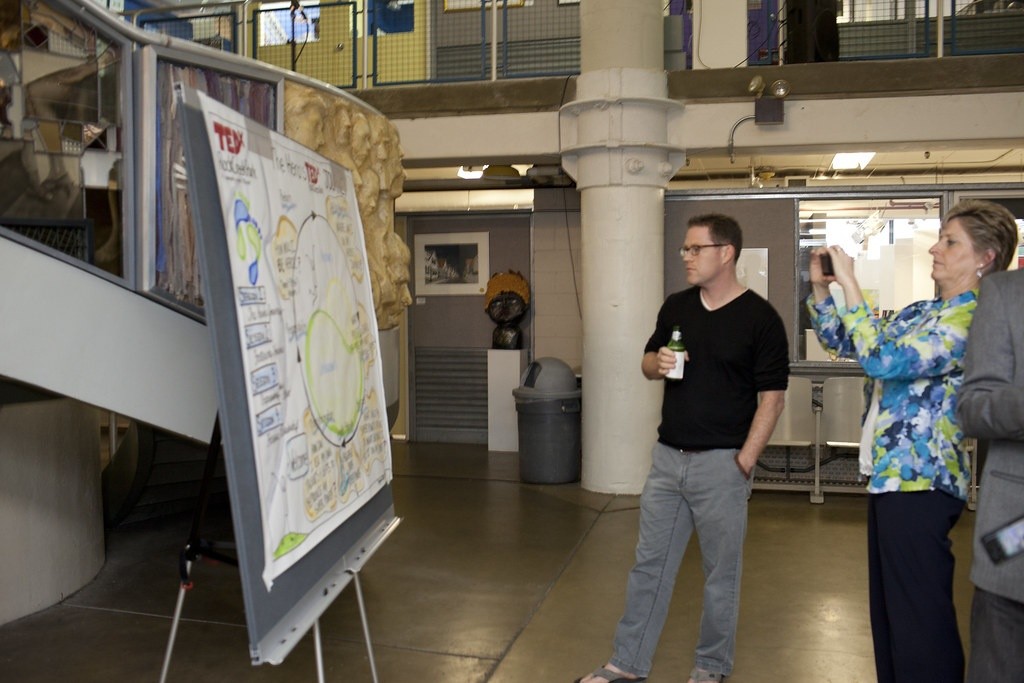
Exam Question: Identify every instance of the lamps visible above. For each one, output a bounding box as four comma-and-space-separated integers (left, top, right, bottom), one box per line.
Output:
747, 72, 790, 123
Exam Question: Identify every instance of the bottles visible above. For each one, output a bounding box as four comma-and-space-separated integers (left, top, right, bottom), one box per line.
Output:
667, 325, 685, 381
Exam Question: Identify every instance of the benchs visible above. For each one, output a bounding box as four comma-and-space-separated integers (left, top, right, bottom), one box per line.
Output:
746, 376, 979, 509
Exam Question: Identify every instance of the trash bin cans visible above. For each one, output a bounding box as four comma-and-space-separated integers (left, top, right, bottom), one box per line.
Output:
510, 356, 583, 485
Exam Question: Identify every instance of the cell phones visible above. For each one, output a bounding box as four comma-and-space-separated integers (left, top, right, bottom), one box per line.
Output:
982, 517, 1024, 565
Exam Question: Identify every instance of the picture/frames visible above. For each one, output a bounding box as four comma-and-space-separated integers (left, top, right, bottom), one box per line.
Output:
413, 230, 489, 295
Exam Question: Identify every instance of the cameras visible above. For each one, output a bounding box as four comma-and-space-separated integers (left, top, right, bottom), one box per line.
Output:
821, 253, 834, 275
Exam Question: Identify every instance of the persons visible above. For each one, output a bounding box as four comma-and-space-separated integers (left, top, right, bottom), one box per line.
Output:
806, 202, 1018, 683
572, 214, 790, 683
955, 266, 1024, 683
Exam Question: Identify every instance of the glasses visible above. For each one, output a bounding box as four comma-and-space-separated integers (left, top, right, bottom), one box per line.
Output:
678, 244, 728, 258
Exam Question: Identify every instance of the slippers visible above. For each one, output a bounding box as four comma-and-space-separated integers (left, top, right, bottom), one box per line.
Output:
575, 665, 647, 683
687, 667, 724, 683
